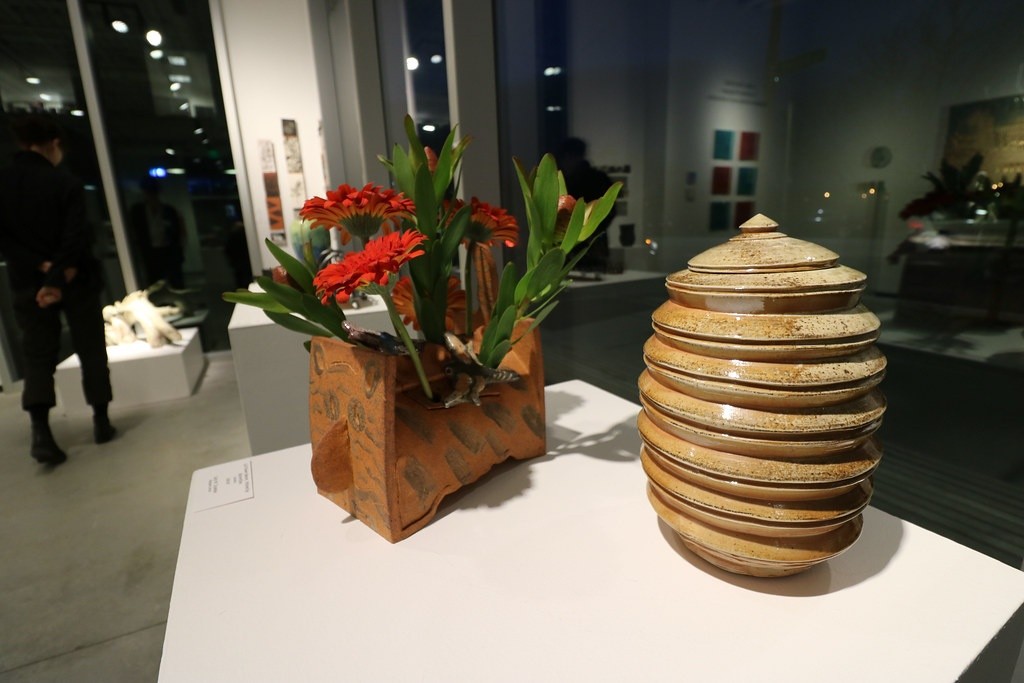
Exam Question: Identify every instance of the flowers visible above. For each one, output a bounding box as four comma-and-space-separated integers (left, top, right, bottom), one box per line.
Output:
221, 114, 624, 412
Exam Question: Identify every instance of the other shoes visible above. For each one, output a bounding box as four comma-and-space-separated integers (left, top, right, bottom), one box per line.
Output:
94, 417, 114, 442
30, 431, 68, 461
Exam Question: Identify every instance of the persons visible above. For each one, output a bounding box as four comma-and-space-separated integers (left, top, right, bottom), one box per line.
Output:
885, 173, 992, 267
557, 136, 620, 273
1, 125, 119, 466
136, 178, 186, 290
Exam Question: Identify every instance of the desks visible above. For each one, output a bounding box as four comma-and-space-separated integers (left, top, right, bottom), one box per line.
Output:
230, 282, 417, 453
154, 380, 1024, 683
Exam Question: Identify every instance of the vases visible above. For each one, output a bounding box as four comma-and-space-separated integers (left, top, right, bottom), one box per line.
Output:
307, 318, 547, 545
